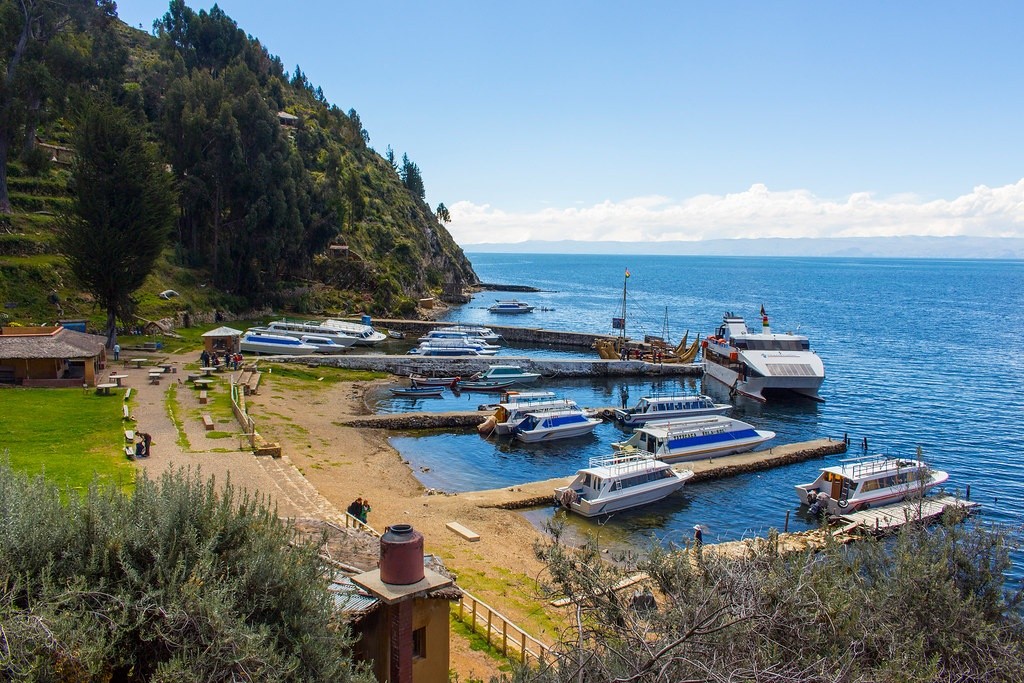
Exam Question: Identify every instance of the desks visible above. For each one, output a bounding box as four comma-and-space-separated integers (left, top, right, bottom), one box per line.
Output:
131, 358, 148, 369
109, 374, 129, 387
187, 364, 224, 390
97, 383, 118, 396
149, 364, 172, 385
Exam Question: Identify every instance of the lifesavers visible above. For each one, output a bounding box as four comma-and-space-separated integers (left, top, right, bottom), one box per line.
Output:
625, 414, 631, 421
483, 376, 487, 381
838, 498, 849, 508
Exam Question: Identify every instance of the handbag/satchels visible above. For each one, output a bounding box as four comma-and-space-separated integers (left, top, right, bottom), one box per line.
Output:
348, 506, 353, 514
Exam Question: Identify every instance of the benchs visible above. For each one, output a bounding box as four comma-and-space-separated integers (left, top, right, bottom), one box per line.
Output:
122, 404, 129, 421
125, 446, 134, 458
126, 429, 133, 443
199, 391, 207, 404
125, 388, 131, 401
202, 415, 214, 430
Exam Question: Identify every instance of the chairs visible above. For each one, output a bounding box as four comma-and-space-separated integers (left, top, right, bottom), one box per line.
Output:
109, 372, 117, 383
82, 383, 92, 396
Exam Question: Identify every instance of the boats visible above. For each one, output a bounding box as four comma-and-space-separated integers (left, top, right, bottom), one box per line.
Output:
702, 310, 826, 404
519, 410, 604, 444
457, 378, 518, 391
490, 299, 536, 313
303, 318, 388, 345
409, 372, 460, 387
613, 390, 734, 424
484, 398, 599, 435
478, 364, 542, 385
554, 450, 694, 518
248, 321, 359, 347
388, 331, 407, 339
480, 391, 557, 410
403, 323, 506, 358
795, 454, 949, 517
241, 330, 319, 356
610, 414, 776, 465
389, 386, 444, 395
590, 266, 700, 363
299, 335, 345, 353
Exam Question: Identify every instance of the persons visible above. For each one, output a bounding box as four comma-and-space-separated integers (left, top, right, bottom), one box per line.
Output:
64, 360, 68, 378
532, 421, 536, 430
135, 432, 151, 457
620, 346, 662, 364
411, 379, 417, 390
88, 324, 154, 336
113, 343, 120, 361
693, 525, 702, 559
620, 382, 629, 409
201, 350, 238, 371
351, 497, 371, 527
